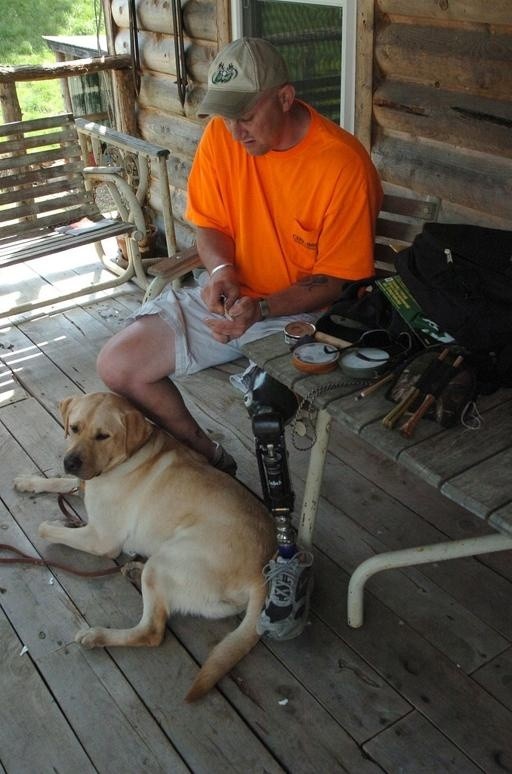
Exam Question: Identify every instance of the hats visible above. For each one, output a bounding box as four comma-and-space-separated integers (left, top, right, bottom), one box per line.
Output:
195, 36, 289, 120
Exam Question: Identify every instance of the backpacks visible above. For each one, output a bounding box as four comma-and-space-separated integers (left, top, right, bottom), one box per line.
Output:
316, 222, 512, 397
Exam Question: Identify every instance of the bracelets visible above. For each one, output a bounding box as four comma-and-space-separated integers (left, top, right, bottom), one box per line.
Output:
210, 260, 235, 278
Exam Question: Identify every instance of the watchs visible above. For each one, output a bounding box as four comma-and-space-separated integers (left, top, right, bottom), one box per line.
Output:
257, 296, 270, 321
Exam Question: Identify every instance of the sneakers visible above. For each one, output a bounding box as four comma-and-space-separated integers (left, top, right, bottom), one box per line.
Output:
211, 441, 238, 475
256, 550, 314, 644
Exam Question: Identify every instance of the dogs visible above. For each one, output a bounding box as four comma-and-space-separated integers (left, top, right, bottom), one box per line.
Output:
12, 391, 281, 703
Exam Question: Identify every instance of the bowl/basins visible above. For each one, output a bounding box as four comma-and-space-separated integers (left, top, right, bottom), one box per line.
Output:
339, 345, 392, 379
290, 341, 340, 375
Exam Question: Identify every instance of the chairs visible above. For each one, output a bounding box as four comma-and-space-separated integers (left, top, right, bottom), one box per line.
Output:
137, 177, 441, 556
0, 115, 178, 330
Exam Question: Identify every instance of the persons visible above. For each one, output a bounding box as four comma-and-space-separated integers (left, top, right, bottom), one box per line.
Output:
98, 40, 387, 641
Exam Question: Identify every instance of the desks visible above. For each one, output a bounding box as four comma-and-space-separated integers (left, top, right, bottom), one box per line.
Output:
241, 324, 512, 628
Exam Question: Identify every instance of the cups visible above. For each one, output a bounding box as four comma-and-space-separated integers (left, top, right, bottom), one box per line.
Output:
283, 320, 317, 348
